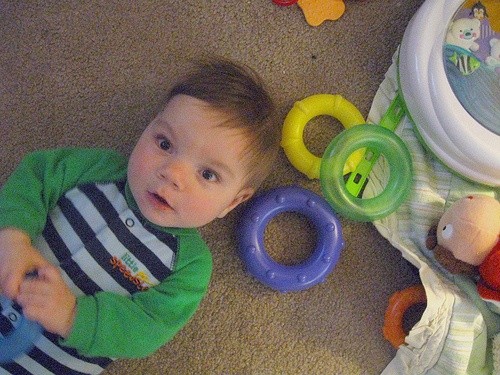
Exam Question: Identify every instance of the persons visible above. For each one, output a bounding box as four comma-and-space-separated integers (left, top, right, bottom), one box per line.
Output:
0, 55, 280, 373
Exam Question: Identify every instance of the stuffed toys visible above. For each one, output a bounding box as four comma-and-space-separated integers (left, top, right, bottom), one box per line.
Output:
426, 193, 500, 303
